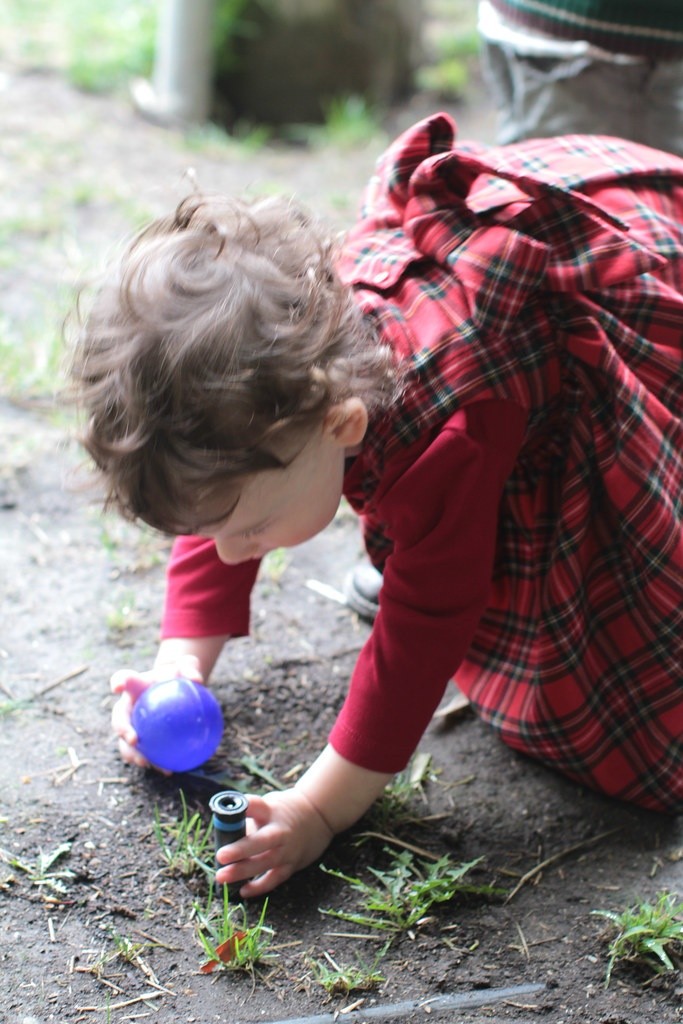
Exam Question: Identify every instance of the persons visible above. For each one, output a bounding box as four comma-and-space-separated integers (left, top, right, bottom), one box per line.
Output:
70, 113, 683, 898
476, 0, 682, 162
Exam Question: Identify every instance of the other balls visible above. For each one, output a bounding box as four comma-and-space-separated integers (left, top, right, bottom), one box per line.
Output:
131, 675, 225, 773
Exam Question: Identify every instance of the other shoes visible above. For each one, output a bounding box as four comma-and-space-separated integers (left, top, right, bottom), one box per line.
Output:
348, 565, 383, 619
